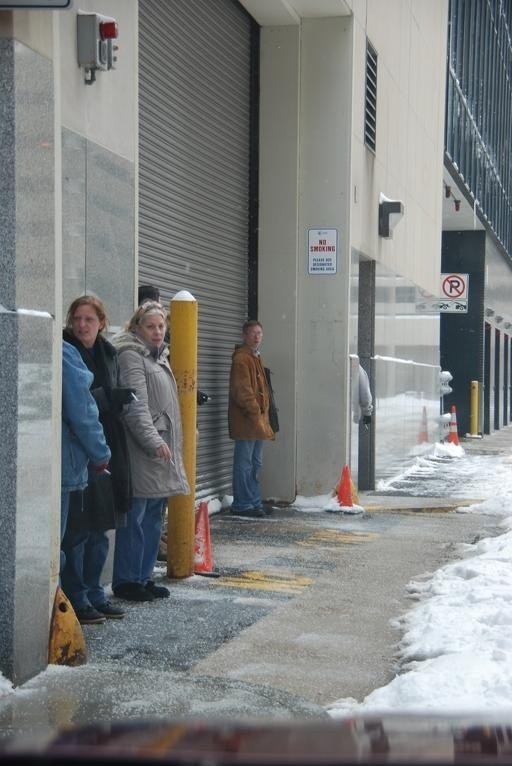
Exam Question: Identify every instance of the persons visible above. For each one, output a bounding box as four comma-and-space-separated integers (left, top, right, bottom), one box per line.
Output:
359, 364, 373, 430
139, 286, 212, 406
229, 321, 279, 516
108, 301, 192, 600
62, 334, 137, 625
61, 294, 112, 625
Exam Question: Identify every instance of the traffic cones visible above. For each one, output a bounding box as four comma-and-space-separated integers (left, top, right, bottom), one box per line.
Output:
192, 501, 221, 578
324, 465, 365, 515
418, 405, 431, 445
446, 406, 462, 446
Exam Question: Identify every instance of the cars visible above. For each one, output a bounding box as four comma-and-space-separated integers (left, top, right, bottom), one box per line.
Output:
2, 708, 511, 764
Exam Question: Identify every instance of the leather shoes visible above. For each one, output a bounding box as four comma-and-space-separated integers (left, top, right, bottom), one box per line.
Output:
232, 506, 274, 517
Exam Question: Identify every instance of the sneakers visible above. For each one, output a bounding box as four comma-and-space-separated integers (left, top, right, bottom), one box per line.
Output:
142, 580, 168, 598
75, 602, 106, 624
90, 598, 124, 618
114, 583, 153, 601
